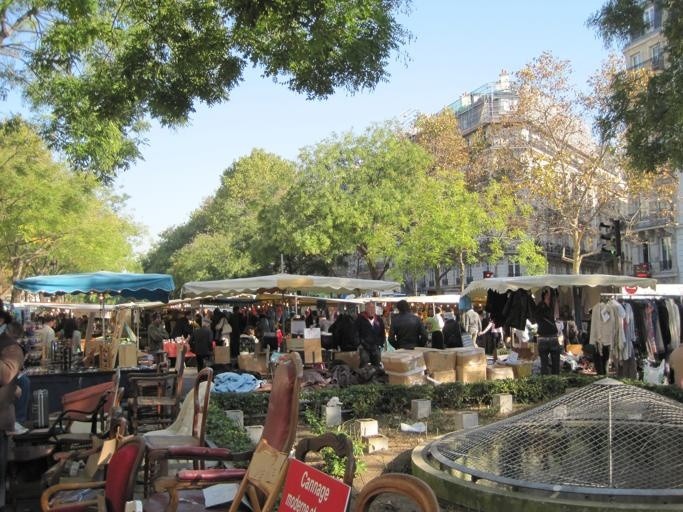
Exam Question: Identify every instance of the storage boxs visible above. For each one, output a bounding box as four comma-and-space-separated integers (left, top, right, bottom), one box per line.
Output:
321, 405, 343, 428
411, 398, 431, 419
416, 346, 532, 383
354, 418, 378, 436
363, 435, 389, 453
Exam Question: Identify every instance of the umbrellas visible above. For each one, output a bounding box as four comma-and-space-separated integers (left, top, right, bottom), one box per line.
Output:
180, 251, 402, 351
456, 269, 657, 305
11, 269, 176, 346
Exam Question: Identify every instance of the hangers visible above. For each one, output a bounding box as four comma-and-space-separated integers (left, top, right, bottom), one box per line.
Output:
597, 292, 683, 304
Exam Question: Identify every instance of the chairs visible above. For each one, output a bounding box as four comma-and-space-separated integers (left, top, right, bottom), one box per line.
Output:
143, 362, 217, 495
130, 347, 186, 431
160, 351, 304, 504
2, 345, 437, 404
63, 368, 122, 434
355, 473, 438, 510
9, 409, 78, 489
644, 358, 665, 384
40, 435, 145, 510
51, 395, 109, 438
290, 430, 356, 510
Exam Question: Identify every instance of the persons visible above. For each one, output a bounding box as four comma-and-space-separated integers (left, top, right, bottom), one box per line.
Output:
0, 297, 612, 378
666, 336, 682, 390
0, 297, 26, 508
533, 285, 560, 376
